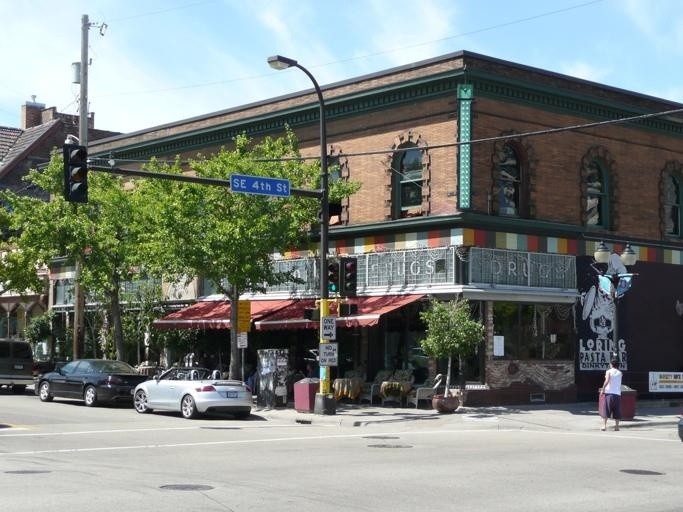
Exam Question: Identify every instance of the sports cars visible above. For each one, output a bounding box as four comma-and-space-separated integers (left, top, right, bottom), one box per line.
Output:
133, 367, 252, 419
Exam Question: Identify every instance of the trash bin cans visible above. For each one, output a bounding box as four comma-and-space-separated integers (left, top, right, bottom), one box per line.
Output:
294, 378, 320, 413
598, 385, 637, 421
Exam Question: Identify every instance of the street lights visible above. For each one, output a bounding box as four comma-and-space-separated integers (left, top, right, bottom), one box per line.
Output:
594, 239, 637, 367
267, 55, 337, 414
64, 133, 79, 145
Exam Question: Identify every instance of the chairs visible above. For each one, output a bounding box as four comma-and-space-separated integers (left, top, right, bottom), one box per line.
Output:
358, 369, 444, 410
191, 369, 221, 380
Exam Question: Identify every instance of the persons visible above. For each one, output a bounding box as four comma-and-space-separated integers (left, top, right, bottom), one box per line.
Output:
598, 358, 623, 431
299, 362, 312, 378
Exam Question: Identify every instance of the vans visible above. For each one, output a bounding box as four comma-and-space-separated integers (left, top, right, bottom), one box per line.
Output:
1, 337, 34, 395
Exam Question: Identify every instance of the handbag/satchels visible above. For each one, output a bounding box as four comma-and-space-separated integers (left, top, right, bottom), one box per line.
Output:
598, 393, 609, 419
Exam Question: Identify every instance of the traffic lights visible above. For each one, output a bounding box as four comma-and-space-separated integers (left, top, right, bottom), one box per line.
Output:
328, 263, 338, 292
345, 262, 355, 291
70, 148, 86, 196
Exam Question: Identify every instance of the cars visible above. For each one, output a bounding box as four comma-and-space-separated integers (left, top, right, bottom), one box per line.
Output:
35, 358, 149, 407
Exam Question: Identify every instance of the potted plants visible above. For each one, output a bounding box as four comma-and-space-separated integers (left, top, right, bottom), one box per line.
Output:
416, 293, 486, 414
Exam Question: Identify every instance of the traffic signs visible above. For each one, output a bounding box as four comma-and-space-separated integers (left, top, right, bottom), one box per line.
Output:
321, 317, 336, 340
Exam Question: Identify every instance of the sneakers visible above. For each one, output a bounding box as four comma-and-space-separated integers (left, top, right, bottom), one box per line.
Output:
615, 428, 619, 431
601, 427, 606, 431
